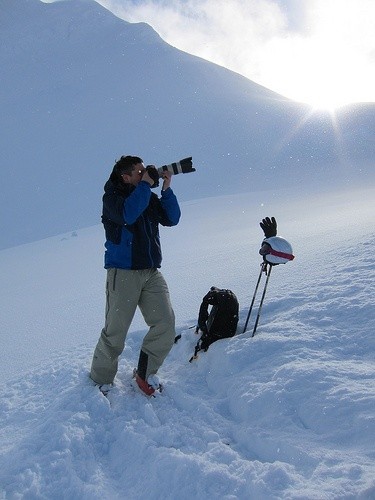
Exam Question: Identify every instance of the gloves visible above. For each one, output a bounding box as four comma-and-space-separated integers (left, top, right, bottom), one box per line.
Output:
260, 216, 278, 238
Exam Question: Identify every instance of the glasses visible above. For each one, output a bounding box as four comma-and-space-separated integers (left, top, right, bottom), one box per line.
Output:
124, 169, 144, 175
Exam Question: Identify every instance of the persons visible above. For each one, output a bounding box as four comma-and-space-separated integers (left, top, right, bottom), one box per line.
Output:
90, 155, 181, 397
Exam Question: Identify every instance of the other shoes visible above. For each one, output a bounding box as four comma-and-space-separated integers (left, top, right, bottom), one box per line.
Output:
133, 368, 164, 397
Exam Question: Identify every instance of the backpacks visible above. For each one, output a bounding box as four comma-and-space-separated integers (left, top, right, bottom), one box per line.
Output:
189, 286, 239, 366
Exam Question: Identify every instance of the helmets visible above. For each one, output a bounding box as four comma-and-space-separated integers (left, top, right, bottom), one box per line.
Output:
259, 236, 296, 266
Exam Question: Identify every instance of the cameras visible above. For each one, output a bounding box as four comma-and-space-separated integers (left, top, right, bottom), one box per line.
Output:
144, 157, 196, 188
